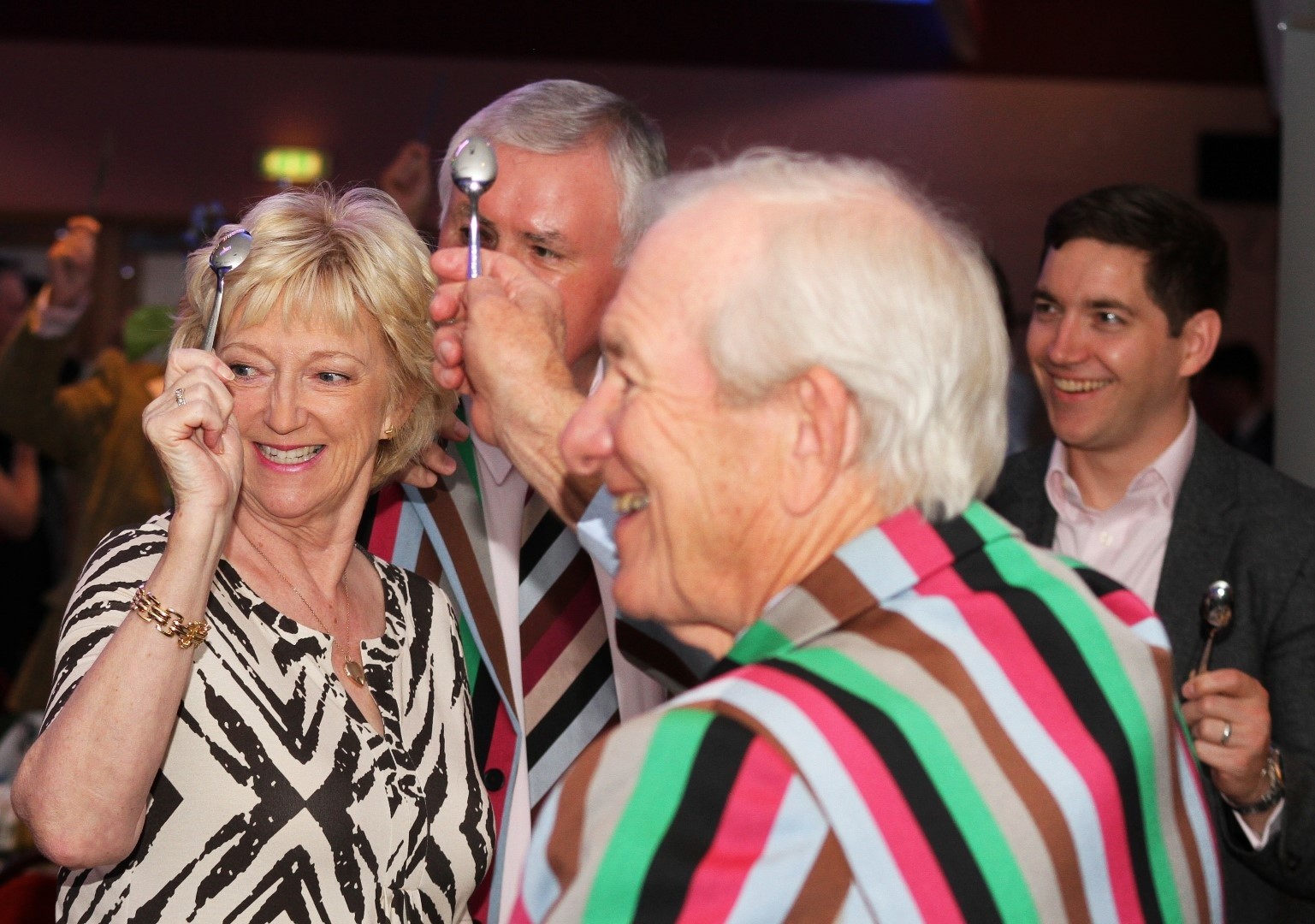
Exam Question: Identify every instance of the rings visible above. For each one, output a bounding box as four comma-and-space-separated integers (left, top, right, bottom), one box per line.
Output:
175, 388, 185, 408
1220, 721, 1234, 744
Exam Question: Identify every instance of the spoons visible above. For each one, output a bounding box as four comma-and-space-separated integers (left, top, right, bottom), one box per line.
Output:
450, 137, 497, 278
199, 229, 253, 351
1196, 580, 1233, 675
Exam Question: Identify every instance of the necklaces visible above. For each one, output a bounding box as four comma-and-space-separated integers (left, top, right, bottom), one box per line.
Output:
231, 513, 368, 687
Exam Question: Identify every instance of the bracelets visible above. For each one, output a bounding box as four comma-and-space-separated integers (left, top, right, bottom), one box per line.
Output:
130, 587, 211, 650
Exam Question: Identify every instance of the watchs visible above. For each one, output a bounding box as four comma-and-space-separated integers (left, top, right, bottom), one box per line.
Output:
1220, 746, 1286, 816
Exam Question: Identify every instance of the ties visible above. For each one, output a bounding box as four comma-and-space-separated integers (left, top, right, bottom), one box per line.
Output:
514, 477, 623, 827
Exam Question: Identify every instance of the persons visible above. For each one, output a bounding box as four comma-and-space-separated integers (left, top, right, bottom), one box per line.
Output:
0, 77, 1315, 924
